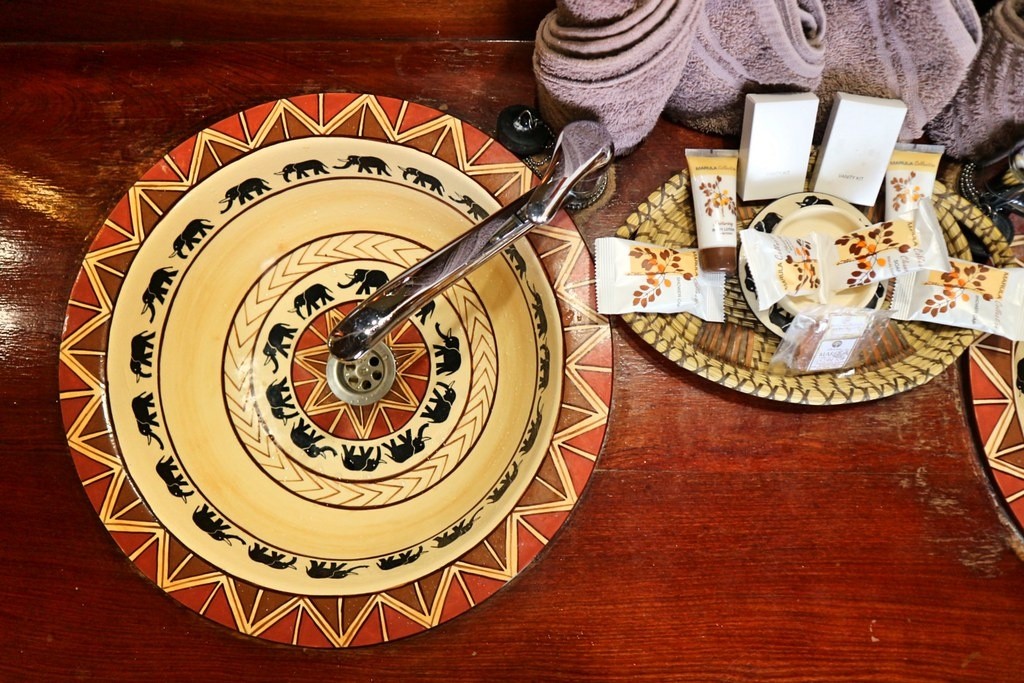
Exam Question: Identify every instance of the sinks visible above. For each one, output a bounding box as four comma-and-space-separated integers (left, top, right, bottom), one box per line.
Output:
57, 91, 614, 650
967, 334, 1024, 535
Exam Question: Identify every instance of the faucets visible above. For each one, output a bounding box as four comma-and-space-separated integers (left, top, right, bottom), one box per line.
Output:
324, 120, 617, 365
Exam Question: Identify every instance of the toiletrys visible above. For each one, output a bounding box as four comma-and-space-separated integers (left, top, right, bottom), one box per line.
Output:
883, 142, 944, 222
681, 147, 738, 273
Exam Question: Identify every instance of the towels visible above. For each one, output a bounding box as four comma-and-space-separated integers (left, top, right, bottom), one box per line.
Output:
534, 0, 1024, 159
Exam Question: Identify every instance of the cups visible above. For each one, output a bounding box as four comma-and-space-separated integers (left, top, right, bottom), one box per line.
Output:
775, 206, 879, 316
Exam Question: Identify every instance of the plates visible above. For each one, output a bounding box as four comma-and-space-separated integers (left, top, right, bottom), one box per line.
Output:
609, 165, 1018, 405
737, 193, 886, 340
